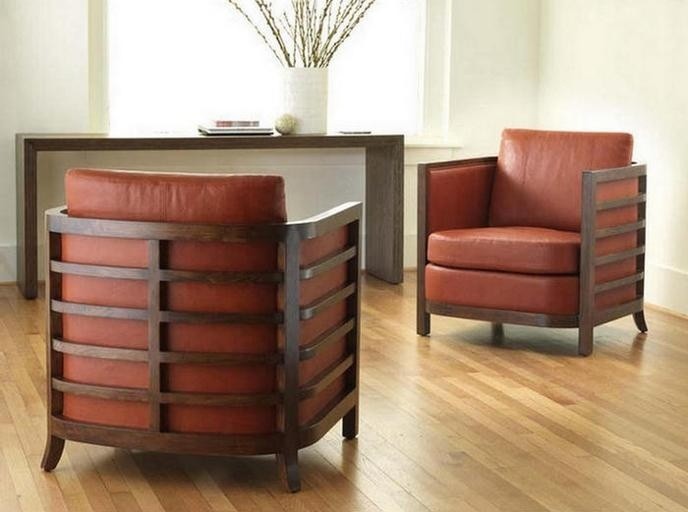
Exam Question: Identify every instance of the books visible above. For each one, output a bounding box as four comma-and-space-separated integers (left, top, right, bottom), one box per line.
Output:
339, 131, 371, 134
196, 119, 274, 136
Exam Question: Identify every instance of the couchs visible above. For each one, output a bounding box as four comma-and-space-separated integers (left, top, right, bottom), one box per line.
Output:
417, 128, 648, 356
40, 167, 361, 493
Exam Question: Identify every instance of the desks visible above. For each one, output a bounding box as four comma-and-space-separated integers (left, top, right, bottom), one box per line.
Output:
16, 132, 405, 300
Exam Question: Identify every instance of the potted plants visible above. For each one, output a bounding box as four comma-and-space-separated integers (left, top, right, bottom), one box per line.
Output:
225, 0, 375, 134
